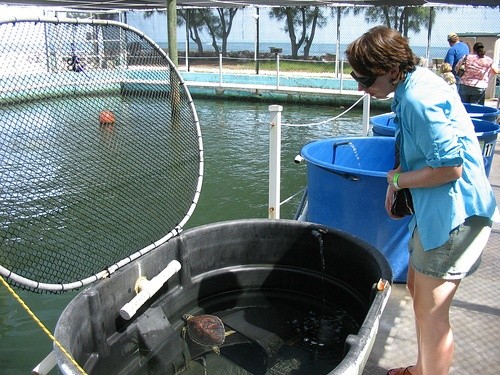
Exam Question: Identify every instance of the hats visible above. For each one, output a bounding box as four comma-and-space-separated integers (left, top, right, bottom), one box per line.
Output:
447, 33, 457, 39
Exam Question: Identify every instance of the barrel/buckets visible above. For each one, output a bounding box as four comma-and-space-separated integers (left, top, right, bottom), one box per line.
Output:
53, 217, 393, 375
300, 136, 488, 284
463, 101, 500, 123
371, 113, 500, 179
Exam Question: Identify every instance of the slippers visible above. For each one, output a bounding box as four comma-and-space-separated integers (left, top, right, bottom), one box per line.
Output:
387, 365, 414, 375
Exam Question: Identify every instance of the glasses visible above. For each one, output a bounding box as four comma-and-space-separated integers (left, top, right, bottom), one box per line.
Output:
350, 71, 377, 88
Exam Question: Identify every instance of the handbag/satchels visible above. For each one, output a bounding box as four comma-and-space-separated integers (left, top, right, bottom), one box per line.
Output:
391, 188, 415, 217
458, 55, 467, 78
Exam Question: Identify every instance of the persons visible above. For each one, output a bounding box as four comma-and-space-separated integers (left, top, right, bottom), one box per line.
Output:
492, 38, 500, 74
444, 34, 469, 81
455, 42, 497, 105
346, 25, 500, 375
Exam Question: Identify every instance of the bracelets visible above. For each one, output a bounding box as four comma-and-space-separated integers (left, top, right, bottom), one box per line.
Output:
392, 173, 401, 190
